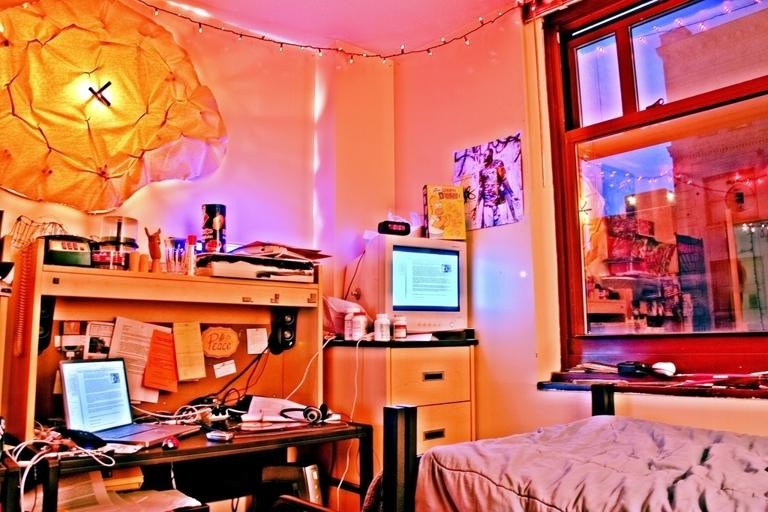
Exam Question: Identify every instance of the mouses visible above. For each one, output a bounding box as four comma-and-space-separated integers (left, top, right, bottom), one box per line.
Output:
161, 436, 181, 449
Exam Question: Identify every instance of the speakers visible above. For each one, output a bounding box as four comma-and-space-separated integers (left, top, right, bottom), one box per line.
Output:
268, 307, 298, 354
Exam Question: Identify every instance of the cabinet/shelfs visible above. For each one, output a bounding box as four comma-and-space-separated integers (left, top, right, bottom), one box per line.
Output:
323, 339, 479, 511
7, 238, 325, 441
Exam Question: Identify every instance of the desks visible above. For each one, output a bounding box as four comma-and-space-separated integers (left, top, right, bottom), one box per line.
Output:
24, 420, 374, 511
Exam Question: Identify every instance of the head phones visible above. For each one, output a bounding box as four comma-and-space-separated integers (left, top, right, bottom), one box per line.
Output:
279, 404, 331, 424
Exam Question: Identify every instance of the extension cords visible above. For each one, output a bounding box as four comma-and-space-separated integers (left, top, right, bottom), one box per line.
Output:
209, 414, 232, 429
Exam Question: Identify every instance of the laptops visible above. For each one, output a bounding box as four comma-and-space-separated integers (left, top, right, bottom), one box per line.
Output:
59, 357, 203, 449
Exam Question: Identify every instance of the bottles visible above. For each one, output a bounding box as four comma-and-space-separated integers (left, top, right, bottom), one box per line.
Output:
344, 308, 407, 342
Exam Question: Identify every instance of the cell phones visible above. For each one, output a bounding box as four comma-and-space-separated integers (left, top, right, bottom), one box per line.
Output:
206, 430, 235, 442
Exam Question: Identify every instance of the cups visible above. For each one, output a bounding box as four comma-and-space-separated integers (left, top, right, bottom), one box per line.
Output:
166, 247, 185, 273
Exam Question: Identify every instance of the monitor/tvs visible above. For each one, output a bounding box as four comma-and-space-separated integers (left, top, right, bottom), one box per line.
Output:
344, 233, 469, 334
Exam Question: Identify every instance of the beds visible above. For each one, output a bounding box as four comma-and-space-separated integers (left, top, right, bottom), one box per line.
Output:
360, 384, 768, 511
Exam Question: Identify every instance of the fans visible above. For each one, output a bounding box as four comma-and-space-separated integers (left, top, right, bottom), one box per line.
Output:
724, 184, 756, 213
